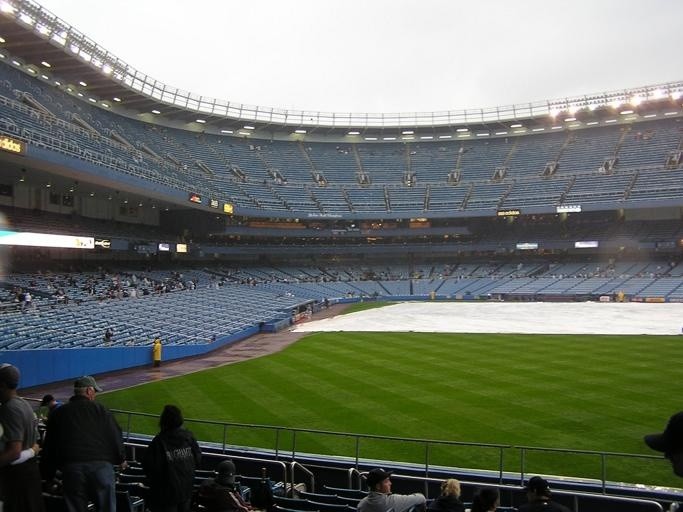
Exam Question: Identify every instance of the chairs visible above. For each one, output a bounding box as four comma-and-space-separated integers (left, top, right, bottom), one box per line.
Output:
0, 59, 683, 350
29, 423, 520, 512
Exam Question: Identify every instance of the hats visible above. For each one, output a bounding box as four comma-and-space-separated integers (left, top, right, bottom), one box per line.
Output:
40, 394, 54, 402
367, 468, 393, 484
218, 460, 236, 485
644, 411, 683, 453
74, 376, 104, 392
0, 364, 20, 389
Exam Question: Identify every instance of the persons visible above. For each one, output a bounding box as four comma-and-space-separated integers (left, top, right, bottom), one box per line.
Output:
469, 486, 501, 512
0, 253, 192, 308
139, 404, 201, 511
249, 481, 276, 512
642, 408, 682, 480
427, 478, 464, 511
196, 460, 267, 512
0, 422, 42, 465
105, 328, 114, 340
354, 468, 426, 511
515, 475, 572, 512
151, 338, 162, 367
193, 249, 682, 289
38, 374, 128, 512
40, 394, 63, 421
0, 362, 45, 511
